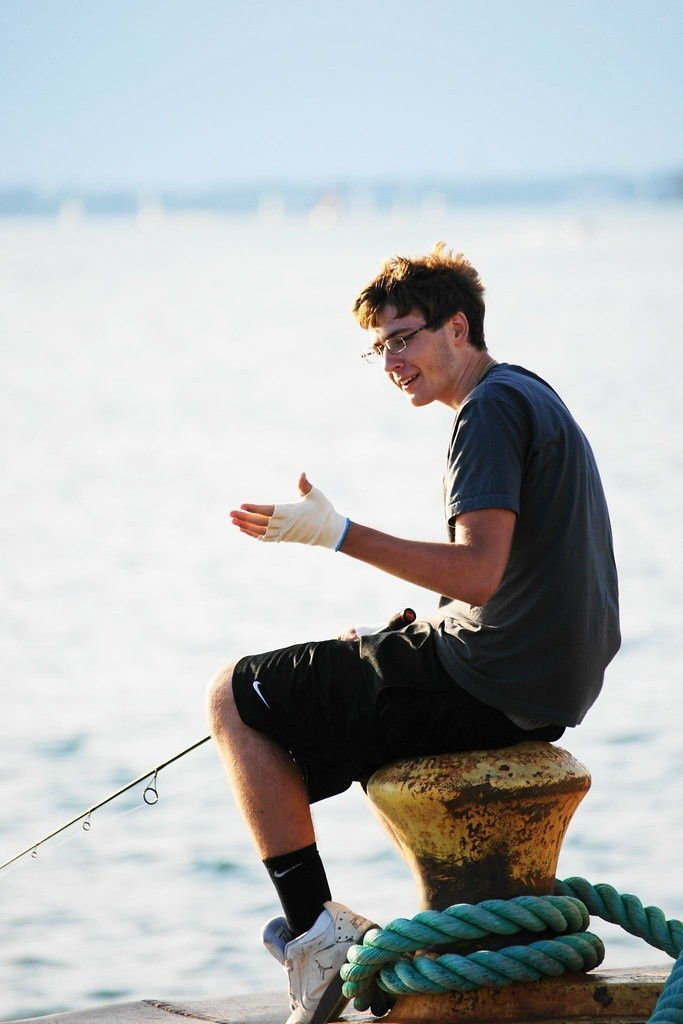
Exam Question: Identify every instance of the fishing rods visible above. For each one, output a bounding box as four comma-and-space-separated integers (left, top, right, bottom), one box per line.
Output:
0, 606, 417, 869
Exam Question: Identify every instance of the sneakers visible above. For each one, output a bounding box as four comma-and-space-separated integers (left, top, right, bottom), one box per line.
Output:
262, 901, 382, 1024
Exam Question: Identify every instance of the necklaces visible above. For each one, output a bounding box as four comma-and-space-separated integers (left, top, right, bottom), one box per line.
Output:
474, 359, 495, 388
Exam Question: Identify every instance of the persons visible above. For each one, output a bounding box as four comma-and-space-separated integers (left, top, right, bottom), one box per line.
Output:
206, 241, 622, 1024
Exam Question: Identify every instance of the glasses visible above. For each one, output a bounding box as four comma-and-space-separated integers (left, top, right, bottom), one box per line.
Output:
361, 324, 430, 365
